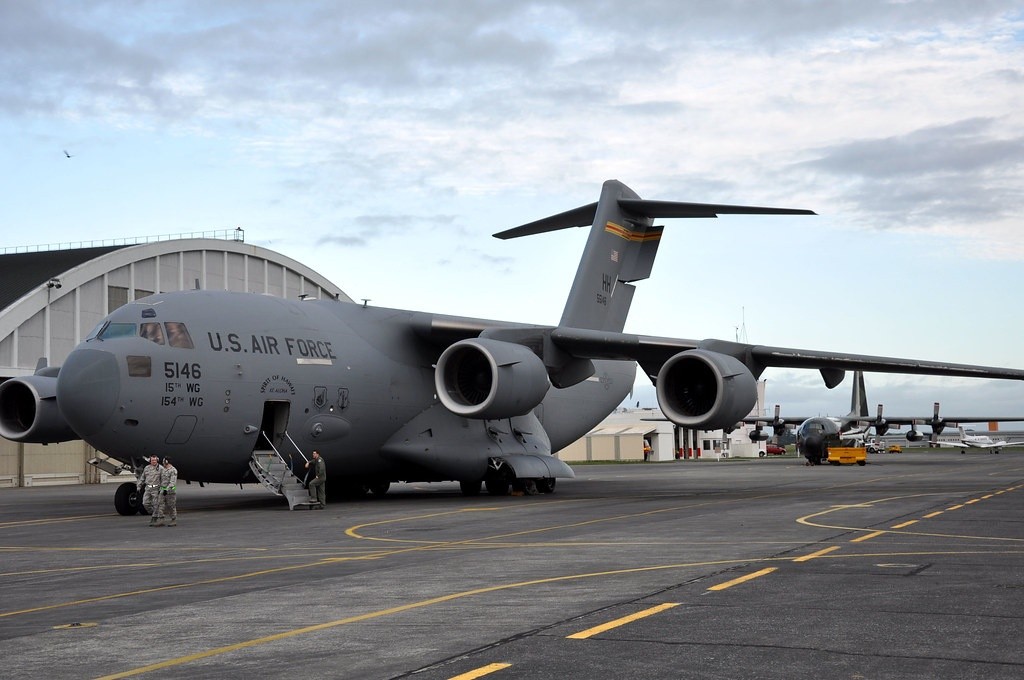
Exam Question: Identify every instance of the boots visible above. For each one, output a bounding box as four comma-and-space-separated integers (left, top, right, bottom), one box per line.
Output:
153, 517, 165, 526
148, 517, 157, 526
167, 518, 176, 527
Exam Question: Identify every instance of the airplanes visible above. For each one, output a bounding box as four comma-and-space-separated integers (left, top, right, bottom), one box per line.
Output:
0, 178, 1024, 512
927, 425, 1024, 454
640, 370, 1024, 466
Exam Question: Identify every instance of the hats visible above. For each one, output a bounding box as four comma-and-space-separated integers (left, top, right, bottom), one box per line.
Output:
164, 456, 171, 463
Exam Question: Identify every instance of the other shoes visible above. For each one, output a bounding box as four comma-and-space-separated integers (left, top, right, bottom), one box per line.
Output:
320, 504, 325, 508
309, 497, 317, 502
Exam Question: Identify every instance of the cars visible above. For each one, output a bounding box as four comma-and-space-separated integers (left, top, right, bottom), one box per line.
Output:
889, 444, 902, 454
766, 444, 787, 455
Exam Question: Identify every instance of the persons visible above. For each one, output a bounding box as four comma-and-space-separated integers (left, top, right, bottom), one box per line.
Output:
305, 449, 326, 508
136, 455, 163, 526
154, 456, 177, 526
